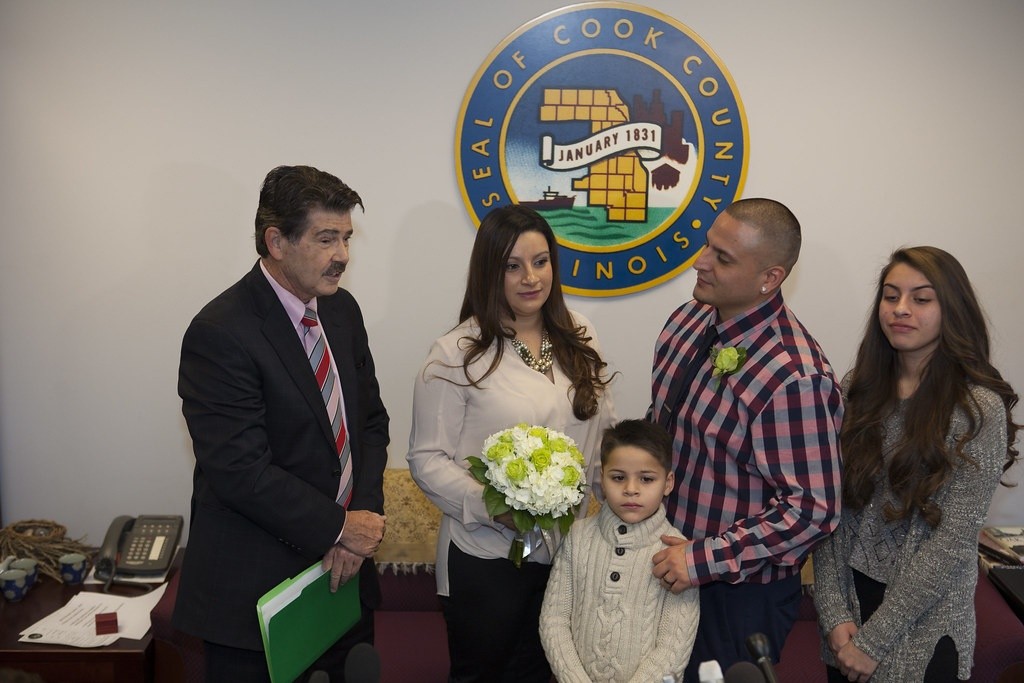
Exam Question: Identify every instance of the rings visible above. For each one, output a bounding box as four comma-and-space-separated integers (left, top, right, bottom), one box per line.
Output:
661, 576, 675, 586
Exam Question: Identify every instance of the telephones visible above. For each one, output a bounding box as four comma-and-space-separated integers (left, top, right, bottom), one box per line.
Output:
95, 513, 185, 585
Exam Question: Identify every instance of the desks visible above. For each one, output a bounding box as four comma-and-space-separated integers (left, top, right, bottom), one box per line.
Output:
0, 545, 176, 683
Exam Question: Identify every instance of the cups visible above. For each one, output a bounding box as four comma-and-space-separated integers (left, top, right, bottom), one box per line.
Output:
58, 553, 87, 586
9, 558, 39, 593
0, 569, 28, 603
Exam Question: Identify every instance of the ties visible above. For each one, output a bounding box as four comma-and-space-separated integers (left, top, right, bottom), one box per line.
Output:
657, 326, 718, 431
300, 308, 354, 511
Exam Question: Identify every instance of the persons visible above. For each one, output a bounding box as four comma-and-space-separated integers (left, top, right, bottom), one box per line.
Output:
537, 419, 702, 683
170, 164, 392, 683
809, 245, 1024, 682
644, 198, 847, 683
405, 205, 620, 683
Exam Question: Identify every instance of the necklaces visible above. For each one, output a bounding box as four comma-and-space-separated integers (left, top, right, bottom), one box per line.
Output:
505, 322, 555, 375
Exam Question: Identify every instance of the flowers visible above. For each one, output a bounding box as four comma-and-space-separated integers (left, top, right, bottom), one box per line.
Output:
461, 426, 588, 569
709, 345, 747, 387
